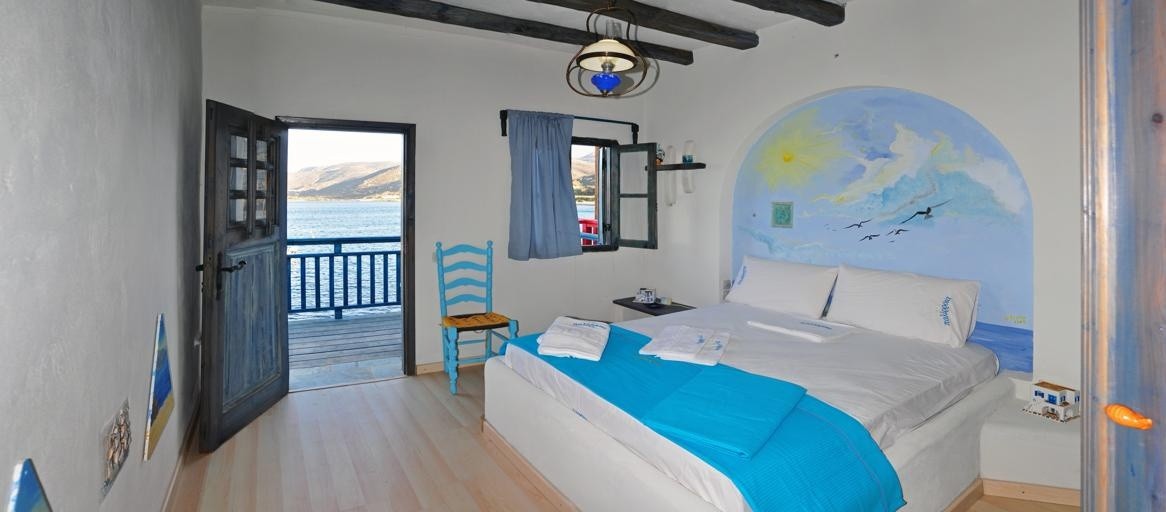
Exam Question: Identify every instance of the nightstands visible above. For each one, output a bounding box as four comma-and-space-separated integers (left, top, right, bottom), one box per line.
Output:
613, 297, 697, 322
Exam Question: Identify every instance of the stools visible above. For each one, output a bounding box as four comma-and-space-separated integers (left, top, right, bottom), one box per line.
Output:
983, 399, 1080, 506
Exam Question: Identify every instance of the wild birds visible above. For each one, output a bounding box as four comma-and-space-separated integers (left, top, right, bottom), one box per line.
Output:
899, 197, 954, 225
844, 219, 871, 229
860, 234, 881, 242
886, 228, 909, 235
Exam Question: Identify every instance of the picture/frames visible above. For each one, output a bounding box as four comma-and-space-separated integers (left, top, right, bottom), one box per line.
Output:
773, 202, 793, 228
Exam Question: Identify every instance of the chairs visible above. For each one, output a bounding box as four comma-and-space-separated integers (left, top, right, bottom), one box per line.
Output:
436, 240, 519, 394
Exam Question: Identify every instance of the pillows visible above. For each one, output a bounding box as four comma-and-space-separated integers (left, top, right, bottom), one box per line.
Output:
824, 265, 980, 348
725, 255, 838, 318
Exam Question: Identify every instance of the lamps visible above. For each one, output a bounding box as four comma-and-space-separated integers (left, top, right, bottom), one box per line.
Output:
565, 1, 648, 97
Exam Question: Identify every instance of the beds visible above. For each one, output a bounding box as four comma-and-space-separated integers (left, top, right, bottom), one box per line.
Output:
483, 303, 1015, 510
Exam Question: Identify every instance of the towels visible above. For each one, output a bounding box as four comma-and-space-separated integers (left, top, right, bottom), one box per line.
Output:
662, 332, 733, 366
639, 324, 714, 357
537, 316, 610, 360
